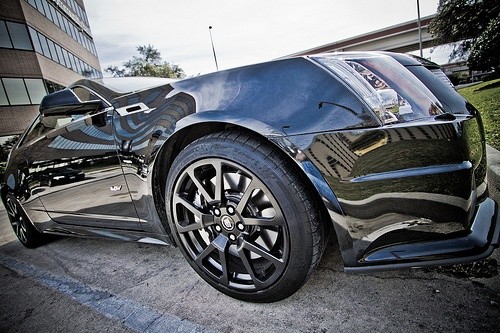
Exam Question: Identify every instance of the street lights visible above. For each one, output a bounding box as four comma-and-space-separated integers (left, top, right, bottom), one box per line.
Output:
208, 26, 219, 71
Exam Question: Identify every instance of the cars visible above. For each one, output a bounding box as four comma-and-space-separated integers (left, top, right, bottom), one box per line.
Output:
0, 51, 498, 302
38, 168, 86, 186
